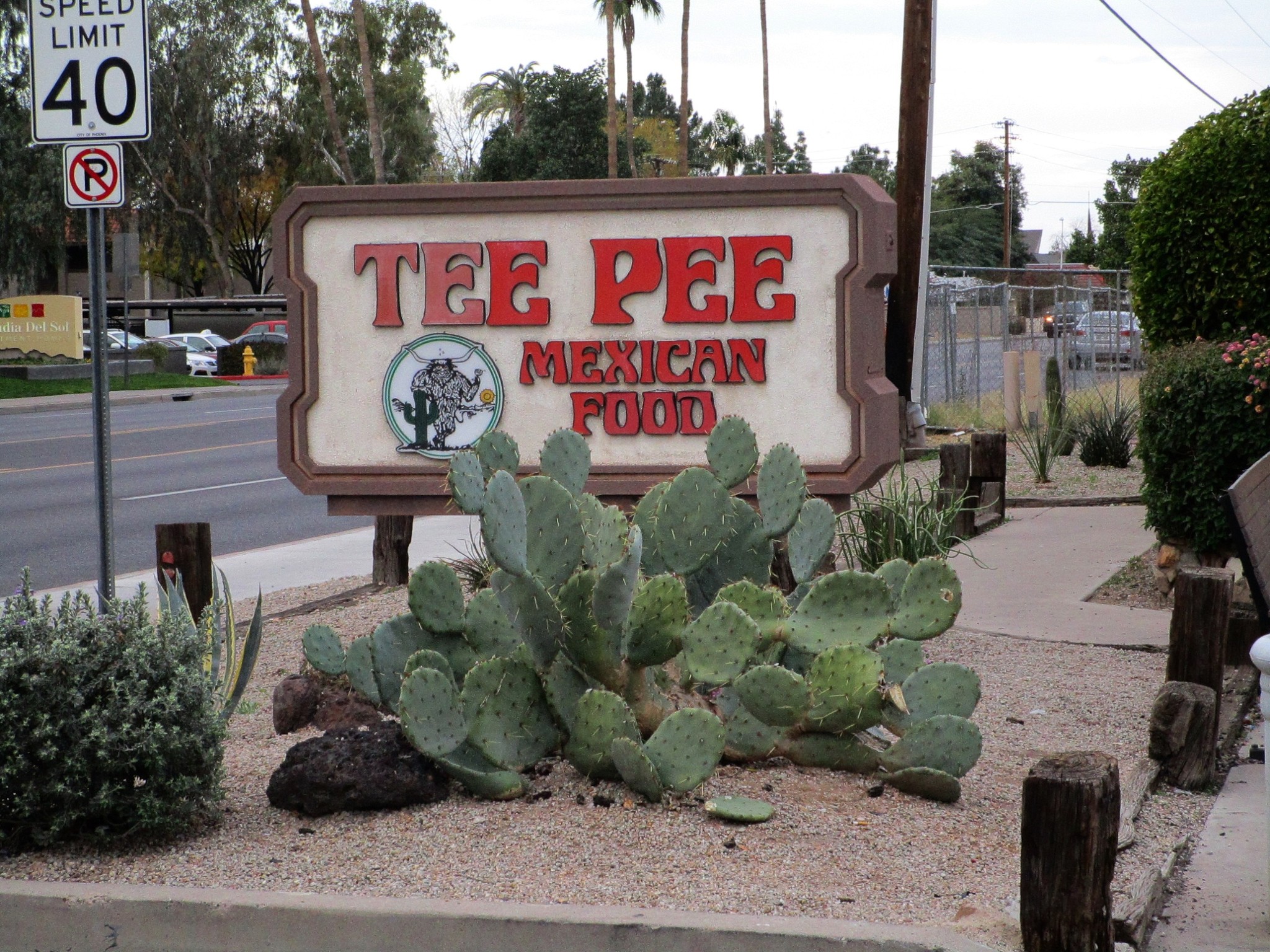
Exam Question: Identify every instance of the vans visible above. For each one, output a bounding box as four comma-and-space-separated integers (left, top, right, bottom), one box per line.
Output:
239, 320, 292, 337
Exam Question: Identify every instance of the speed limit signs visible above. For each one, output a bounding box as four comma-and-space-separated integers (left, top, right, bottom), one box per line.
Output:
27, 1, 152, 145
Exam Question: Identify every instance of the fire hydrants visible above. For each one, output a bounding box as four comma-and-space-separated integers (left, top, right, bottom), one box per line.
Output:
242, 346, 258, 377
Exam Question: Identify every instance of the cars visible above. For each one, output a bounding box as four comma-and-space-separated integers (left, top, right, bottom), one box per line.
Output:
1070, 310, 1145, 367
214, 332, 290, 375
142, 338, 218, 377
158, 329, 231, 363
83, 330, 146, 354
1043, 302, 1090, 338
